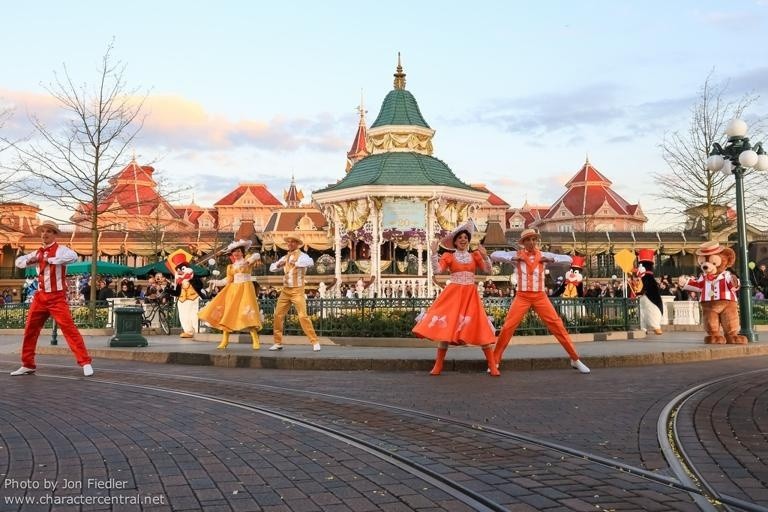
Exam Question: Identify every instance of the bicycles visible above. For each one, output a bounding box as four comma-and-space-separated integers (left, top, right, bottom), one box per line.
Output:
126, 293, 171, 335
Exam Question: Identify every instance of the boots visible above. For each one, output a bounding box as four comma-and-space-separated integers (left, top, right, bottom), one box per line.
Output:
250, 330, 260, 350
482, 346, 501, 376
217, 330, 228, 349
429, 348, 447, 375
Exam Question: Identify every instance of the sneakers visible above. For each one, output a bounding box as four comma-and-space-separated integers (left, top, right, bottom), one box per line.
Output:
11, 366, 36, 376
571, 358, 591, 374
313, 343, 321, 352
84, 364, 94, 376
269, 345, 283, 351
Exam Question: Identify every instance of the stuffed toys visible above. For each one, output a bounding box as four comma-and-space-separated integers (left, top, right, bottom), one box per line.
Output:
163, 261, 208, 338
549, 254, 589, 325
623, 249, 664, 335
679, 241, 748, 345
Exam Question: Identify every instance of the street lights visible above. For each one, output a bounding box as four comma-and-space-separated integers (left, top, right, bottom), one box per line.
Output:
704, 117, 768, 344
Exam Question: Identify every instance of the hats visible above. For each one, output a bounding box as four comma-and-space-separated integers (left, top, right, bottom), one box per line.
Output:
36, 220, 61, 234
518, 228, 541, 244
440, 222, 475, 252
283, 233, 304, 245
226, 239, 254, 252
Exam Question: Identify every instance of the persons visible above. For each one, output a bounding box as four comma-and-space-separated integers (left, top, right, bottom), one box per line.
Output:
9, 220, 95, 376
195, 240, 264, 351
0, 271, 768, 326
268, 236, 320, 351
488, 229, 591, 374
411, 218, 500, 378
